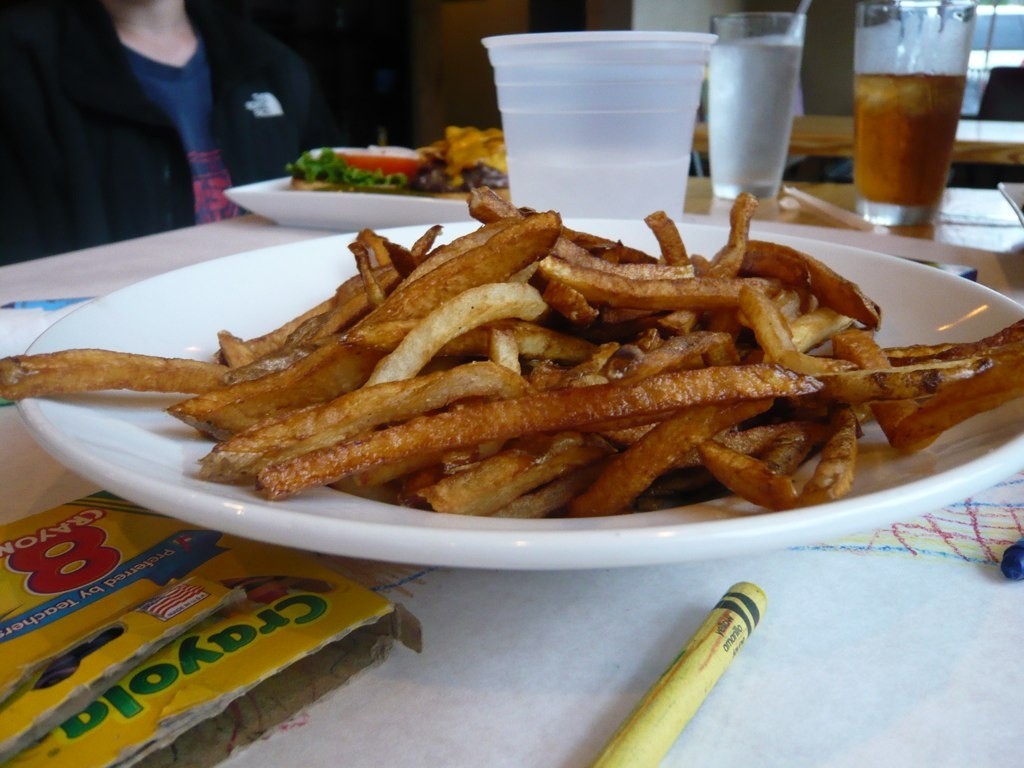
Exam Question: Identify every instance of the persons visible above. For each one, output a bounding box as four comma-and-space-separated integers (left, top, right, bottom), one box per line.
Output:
0, 0, 313, 259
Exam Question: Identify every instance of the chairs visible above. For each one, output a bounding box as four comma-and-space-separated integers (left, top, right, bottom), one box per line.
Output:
978, 67, 1024, 120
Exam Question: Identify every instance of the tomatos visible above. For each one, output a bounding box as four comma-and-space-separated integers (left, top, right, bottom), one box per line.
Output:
341, 154, 422, 177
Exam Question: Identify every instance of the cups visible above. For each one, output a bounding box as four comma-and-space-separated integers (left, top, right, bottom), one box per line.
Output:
480, 31, 721, 223
706, 13, 807, 199
851, 1, 976, 226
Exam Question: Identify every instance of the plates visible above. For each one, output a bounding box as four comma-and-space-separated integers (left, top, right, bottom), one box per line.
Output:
223, 176, 472, 233
18, 221, 1024, 571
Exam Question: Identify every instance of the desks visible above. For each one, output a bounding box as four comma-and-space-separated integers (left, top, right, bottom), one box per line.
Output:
0, 114, 1024, 768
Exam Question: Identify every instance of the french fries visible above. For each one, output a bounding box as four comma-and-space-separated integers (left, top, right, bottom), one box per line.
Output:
0, 182, 1024, 512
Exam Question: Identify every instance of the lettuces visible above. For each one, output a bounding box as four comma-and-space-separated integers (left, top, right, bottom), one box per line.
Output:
286, 148, 407, 188
